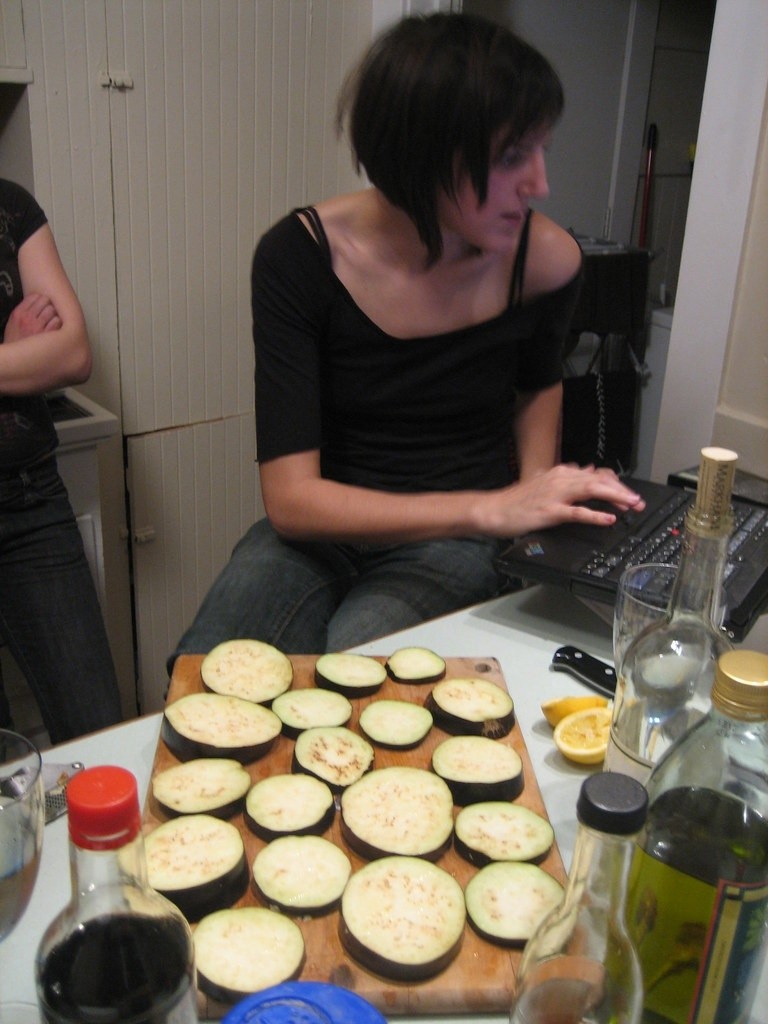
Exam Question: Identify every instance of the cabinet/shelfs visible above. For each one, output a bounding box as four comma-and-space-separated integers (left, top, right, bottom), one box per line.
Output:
0, 0, 468, 751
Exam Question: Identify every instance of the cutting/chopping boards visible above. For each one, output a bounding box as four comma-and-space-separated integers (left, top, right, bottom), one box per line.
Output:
144, 653, 603, 1019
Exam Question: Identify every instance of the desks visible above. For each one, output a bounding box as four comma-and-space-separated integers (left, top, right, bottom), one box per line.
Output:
0, 582, 768, 1024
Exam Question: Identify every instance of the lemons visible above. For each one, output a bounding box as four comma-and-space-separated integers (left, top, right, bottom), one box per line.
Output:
554, 707, 612, 765
541, 696, 608, 728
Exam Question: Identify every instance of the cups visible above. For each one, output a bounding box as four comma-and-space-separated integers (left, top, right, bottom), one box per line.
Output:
0, 728, 44, 940
613, 565, 729, 679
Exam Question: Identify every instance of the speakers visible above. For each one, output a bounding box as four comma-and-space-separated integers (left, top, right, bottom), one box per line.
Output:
562, 238, 654, 473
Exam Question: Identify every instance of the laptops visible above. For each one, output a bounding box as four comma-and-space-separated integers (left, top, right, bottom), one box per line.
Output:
502, 472, 768, 643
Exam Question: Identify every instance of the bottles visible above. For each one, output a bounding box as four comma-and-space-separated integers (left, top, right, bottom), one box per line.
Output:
605, 447, 739, 791
35, 766, 198, 1024
605, 650, 767, 1024
513, 774, 643, 1024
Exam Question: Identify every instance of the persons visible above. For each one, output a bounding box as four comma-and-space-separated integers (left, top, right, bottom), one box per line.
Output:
166, 13, 648, 680
0, 177, 125, 747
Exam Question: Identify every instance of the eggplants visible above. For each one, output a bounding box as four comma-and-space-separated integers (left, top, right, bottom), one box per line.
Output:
117, 636, 565, 1003
